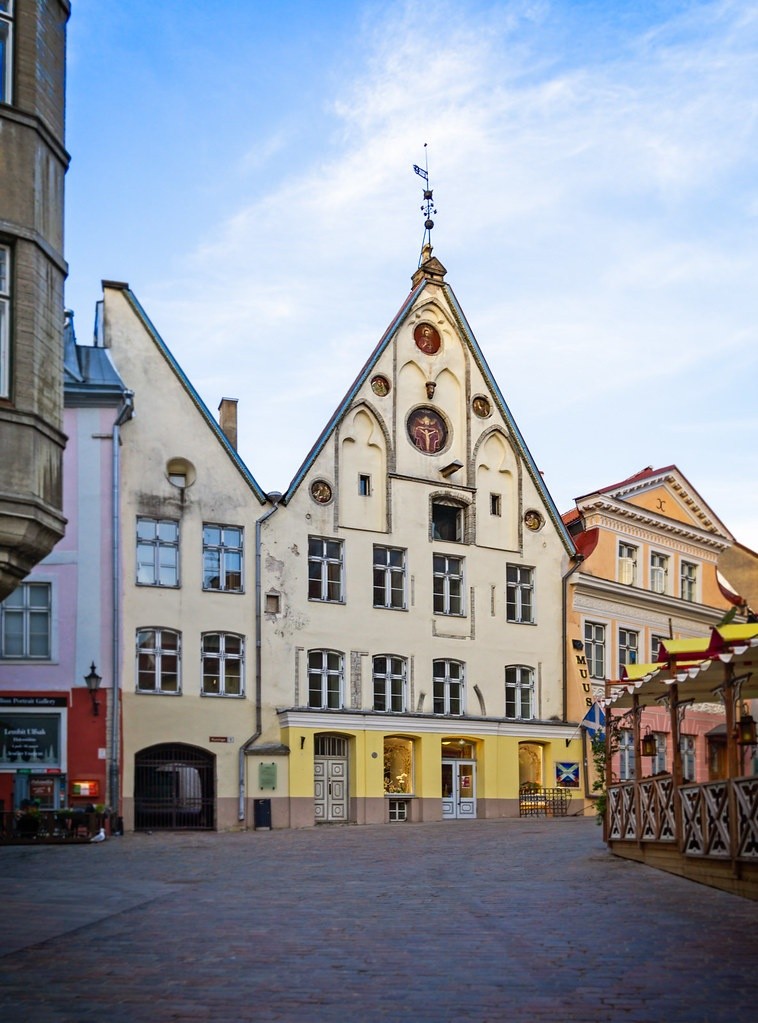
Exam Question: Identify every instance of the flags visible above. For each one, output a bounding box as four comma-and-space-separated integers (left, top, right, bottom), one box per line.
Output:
583, 701, 607, 742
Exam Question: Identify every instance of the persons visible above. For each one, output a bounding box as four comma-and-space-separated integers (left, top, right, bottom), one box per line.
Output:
313, 484, 330, 501
526, 514, 538, 529
418, 326, 434, 353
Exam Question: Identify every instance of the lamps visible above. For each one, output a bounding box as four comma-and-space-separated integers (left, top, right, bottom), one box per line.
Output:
83, 661, 103, 715
640, 725, 658, 756
735, 702, 758, 746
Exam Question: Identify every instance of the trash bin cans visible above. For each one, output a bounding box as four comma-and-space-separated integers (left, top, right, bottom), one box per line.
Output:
254, 798, 272, 832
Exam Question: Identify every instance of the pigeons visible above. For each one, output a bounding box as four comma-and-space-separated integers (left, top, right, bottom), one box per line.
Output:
89, 828, 106, 844
